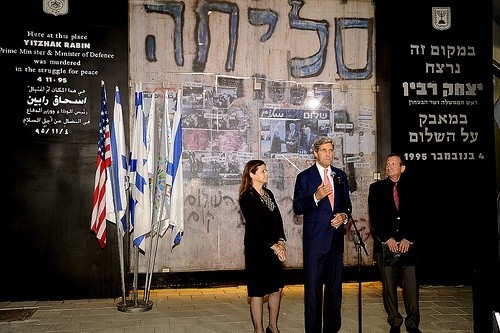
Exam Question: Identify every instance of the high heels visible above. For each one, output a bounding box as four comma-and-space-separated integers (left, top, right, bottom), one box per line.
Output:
266, 326, 280, 333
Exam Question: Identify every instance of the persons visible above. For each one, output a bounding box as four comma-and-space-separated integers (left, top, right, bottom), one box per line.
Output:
237, 160, 287, 333
293, 137, 353, 333
368, 152, 423, 333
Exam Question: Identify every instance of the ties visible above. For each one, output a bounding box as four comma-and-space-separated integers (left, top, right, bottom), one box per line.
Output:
324, 168, 334, 212
393, 183, 399, 210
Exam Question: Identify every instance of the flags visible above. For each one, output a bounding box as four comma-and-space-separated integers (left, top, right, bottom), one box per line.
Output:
89, 83, 113, 250
120, 92, 185, 255
107, 89, 128, 238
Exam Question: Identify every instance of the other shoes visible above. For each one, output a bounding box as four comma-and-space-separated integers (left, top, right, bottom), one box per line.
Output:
389, 326, 400, 333
406, 325, 422, 333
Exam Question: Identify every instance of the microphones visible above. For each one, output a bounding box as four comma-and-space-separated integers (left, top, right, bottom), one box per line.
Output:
330, 171, 340, 185
